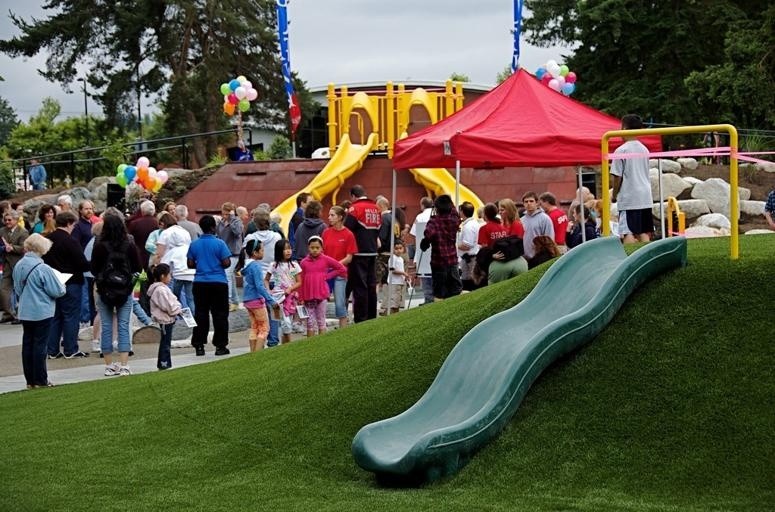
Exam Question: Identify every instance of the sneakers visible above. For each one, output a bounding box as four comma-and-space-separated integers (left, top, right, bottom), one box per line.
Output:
26, 340, 230, 389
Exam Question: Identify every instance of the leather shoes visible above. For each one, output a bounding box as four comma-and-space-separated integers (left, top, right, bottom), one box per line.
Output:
0, 318, 21, 324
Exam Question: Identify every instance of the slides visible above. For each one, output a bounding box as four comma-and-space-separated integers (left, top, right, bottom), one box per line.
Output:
353, 235, 686, 476
410, 168, 485, 216
269, 133, 378, 239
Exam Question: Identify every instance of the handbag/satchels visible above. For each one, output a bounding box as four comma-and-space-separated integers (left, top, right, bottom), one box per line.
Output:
492, 235, 524, 264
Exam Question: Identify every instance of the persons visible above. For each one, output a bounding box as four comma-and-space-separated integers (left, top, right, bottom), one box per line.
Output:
764, 189, 775, 230
89, 206, 143, 376
410, 183, 639, 302
0, 184, 410, 361
609, 112, 656, 244
11, 232, 68, 389
28, 158, 47, 190
147, 262, 185, 372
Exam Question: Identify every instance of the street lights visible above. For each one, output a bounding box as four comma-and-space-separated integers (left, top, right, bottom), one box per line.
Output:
77, 77, 88, 143
16, 145, 32, 191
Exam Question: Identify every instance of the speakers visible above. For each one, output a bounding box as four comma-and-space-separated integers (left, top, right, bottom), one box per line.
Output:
107, 184, 126, 216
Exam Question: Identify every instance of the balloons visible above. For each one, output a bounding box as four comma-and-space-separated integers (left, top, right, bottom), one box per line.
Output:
219, 73, 258, 117
113, 155, 170, 198
534, 58, 578, 99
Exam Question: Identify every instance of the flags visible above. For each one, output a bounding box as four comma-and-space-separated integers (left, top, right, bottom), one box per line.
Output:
510, 0, 524, 74
276, 0, 303, 132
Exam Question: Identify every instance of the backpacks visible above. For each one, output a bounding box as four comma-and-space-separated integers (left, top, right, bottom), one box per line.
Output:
95, 236, 138, 306
